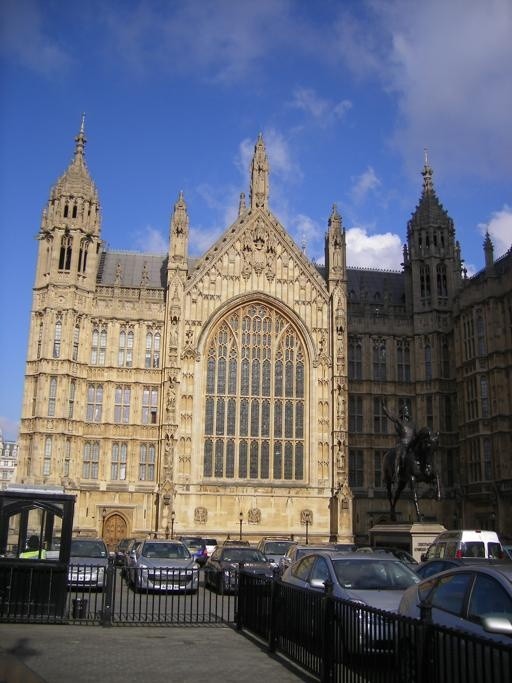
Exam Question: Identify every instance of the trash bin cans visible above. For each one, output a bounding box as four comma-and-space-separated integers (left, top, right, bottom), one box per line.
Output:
72, 598, 87, 619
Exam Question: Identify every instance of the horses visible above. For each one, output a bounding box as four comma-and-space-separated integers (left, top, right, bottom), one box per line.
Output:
382, 427, 443, 524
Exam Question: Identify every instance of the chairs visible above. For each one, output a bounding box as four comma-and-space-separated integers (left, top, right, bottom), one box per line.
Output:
168, 548, 178, 558
146, 545, 156, 558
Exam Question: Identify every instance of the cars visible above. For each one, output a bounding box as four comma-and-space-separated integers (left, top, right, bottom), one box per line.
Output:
201, 538, 418, 595
115, 539, 200, 592
47, 535, 109, 590
281, 550, 422, 655
398, 559, 512, 682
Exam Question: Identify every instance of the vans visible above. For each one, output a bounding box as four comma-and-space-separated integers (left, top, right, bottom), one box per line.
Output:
425, 529, 503, 558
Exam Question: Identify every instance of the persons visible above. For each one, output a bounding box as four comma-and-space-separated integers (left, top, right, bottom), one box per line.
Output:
382, 403, 417, 483
18, 535, 47, 559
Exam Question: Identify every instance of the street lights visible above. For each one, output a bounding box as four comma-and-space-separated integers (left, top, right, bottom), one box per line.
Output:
369, 515, 374, 545
239, 512, 243, 539
304, 514, 310, 545
171, 512, 174, 538
